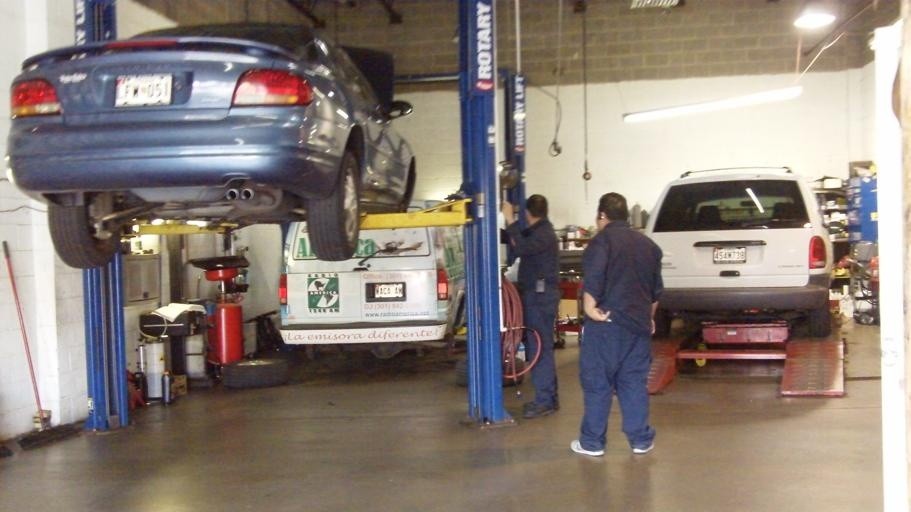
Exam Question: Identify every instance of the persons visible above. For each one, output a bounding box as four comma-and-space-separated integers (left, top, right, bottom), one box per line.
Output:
501, 195, 562, 419
571, 192, 664, 457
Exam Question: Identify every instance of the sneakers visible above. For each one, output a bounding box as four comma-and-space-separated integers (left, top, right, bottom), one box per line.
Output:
632, 442, 655, 453
570, 439, 605, 456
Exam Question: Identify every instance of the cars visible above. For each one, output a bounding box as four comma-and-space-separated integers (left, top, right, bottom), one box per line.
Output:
6, 22, 417, 269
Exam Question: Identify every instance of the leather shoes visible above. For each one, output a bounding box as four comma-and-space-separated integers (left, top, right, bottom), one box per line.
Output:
522, 397, 558, 418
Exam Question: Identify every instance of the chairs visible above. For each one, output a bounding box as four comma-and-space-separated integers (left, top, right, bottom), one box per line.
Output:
696, 200, 799, 223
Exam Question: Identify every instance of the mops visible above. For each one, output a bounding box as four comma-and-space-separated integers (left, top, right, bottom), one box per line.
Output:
1, 240, 79, 452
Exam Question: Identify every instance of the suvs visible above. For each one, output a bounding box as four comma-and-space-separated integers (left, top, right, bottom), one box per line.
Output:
643, 165, 835, 337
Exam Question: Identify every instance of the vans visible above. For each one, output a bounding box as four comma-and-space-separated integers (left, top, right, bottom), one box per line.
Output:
278, 199, 466, 378
